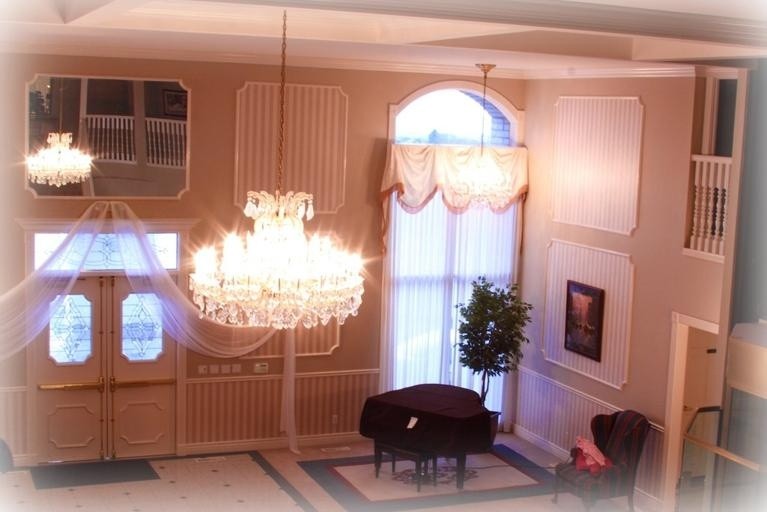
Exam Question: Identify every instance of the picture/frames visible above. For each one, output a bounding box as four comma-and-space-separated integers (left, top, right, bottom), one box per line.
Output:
564, 279, 605, 361
163, 89, 187, 117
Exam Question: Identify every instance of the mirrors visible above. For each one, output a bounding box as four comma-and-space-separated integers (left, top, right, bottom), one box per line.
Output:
23, 73, 192, 201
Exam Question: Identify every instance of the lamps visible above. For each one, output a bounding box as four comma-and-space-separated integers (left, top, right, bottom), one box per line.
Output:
27, 77, 92, 188
186, 10, 363, 331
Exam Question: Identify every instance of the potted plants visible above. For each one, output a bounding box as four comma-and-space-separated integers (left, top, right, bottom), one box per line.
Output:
452, 275, 533, 453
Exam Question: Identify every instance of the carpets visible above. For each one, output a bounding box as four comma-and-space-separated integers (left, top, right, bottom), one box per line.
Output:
30, 459, 160, 489
297, 443, 563, 512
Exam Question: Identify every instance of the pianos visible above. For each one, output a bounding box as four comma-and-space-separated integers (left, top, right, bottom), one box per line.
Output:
360, 383, 502, 493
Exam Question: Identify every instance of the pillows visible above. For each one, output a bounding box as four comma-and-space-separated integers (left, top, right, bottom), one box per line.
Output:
576, 451, 613, 473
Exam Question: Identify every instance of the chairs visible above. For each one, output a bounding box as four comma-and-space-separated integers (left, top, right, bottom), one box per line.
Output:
552, 409, 651, 512
